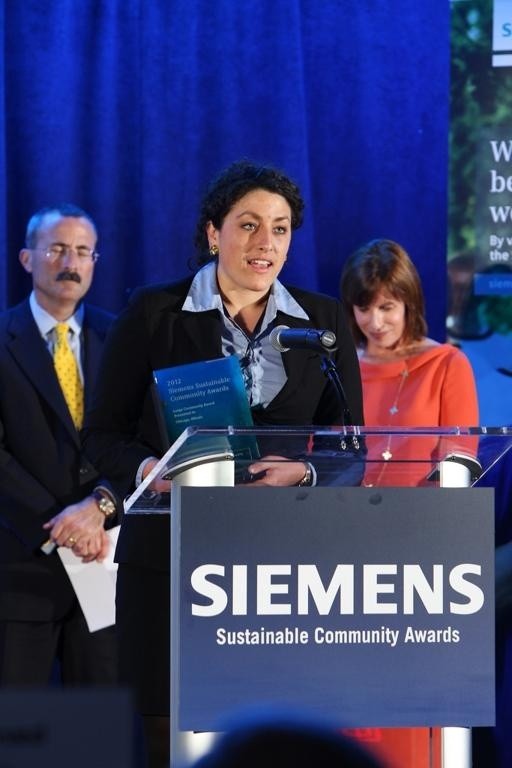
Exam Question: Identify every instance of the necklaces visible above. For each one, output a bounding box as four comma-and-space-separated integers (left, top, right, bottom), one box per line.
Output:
361, 338, 418, 487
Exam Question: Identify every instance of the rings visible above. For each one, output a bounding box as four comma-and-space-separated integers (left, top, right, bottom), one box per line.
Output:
69, 538, 77, 543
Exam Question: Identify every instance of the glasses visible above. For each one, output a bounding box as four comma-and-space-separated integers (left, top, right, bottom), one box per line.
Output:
24, 245, 102, 264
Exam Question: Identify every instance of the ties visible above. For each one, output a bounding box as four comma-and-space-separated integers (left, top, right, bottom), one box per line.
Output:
50, 320, 87, 433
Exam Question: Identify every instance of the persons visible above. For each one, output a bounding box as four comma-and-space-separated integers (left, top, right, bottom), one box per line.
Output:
70, 161, 366, 713
1, 201, 149, 683
329, 237, 482, 768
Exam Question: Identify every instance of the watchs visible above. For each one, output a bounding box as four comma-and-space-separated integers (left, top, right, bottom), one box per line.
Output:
91, 491, 118, 517
298, 462, 312, 486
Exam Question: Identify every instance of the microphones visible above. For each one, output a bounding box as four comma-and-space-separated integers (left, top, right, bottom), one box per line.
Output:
269, 324, 337, 353
